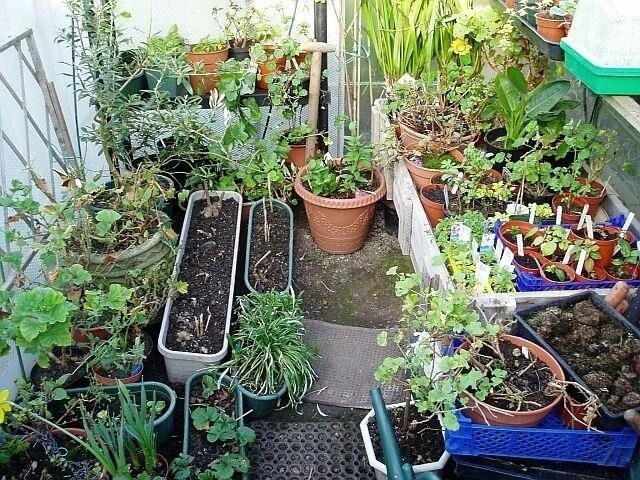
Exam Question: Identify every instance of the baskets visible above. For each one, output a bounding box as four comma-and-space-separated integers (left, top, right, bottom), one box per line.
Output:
493, 210, 640, 293
439, 330, 638, 469
